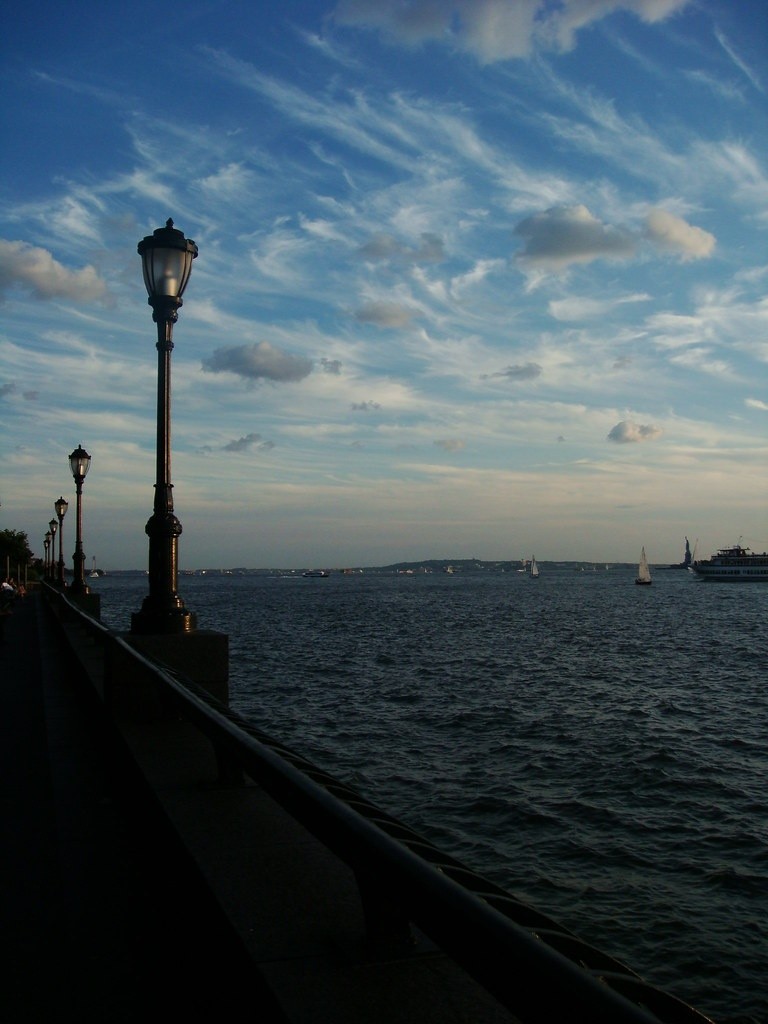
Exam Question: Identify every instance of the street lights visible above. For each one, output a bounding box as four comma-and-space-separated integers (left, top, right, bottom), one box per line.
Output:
43, 539, 50, 578
49, 517, 58, 582
68, 444, 91, 597
54, 496, 68, 587
130, 217, 199, 641
45, 530, 53, 578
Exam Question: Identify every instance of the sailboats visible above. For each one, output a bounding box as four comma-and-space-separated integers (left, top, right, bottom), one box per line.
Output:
528, 554, 540, 579
635, 545, 652, 585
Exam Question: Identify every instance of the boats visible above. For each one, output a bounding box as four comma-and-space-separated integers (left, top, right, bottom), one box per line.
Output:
670, 535, 768, 583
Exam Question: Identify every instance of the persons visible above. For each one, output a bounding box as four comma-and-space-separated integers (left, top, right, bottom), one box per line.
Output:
0, 577, 18, 598
19, 581, 33, 598
